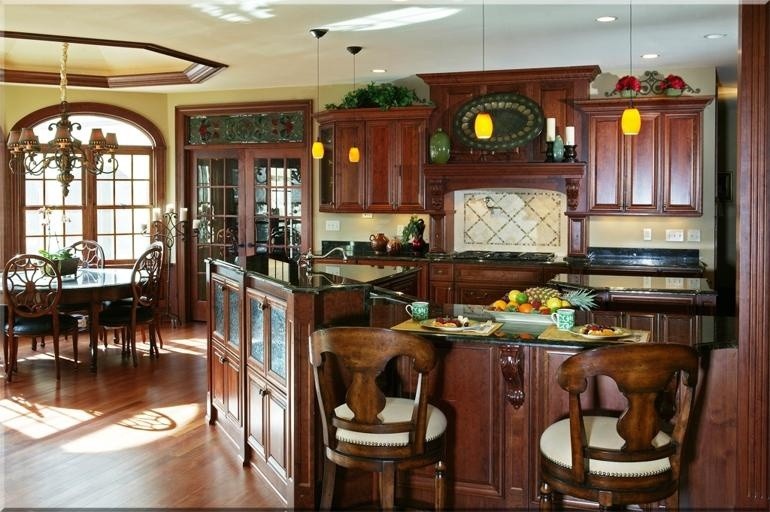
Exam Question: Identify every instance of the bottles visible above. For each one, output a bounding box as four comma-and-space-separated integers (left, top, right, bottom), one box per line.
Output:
553, 135, 563, 161
430, 128, 450, 165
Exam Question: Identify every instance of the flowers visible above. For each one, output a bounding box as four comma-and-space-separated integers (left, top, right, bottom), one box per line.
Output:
36, 202, 77, 274
660, 73, 684, 89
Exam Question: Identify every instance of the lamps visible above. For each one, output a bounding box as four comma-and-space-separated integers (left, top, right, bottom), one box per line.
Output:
6, 43, 119, 196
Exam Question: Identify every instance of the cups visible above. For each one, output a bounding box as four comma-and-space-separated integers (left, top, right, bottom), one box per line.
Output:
551, 309, 576, 331
404, 301, 428, 322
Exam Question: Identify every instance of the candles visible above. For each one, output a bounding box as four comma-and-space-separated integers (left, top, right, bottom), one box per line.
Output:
193, 218, 201, 229
153, 207, 161, 221
179, 208, 188, 222
166, 204, 174, 213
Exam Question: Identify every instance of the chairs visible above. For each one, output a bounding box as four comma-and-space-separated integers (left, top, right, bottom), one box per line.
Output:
0, 241, 168, 386
538, 337, 696, 510
302, 325, 452, 508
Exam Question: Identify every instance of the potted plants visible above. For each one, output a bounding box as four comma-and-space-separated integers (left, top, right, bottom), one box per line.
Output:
400, 216, 428, 255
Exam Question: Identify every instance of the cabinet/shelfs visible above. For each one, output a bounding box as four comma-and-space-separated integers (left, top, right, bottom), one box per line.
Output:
244, 276, 371, 504
427, 263, 561, 312
589, 309, 702, 345
202, 264, 247, 472
315, 105, 433, 214
571, 95, 711, 220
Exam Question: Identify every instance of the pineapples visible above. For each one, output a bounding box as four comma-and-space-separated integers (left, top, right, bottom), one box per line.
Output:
524, 287, 600, 312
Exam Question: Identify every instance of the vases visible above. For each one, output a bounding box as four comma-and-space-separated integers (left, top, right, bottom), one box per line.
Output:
665, 88, 682, 97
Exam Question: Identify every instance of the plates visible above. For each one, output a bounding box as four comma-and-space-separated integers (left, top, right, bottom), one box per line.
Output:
570, 325, 630, 339
421, 318, 479, 331
455, 93, 544, 151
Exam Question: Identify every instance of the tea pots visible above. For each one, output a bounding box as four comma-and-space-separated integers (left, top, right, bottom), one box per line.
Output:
369, 234, 401, 255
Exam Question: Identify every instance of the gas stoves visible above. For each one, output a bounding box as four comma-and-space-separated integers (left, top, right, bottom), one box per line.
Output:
455, 251, 554, 263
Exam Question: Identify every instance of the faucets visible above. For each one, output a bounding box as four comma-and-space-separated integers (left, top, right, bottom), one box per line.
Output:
301, 247, 348, 272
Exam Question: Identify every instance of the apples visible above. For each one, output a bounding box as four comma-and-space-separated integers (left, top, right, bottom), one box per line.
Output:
493, 290, 571, 315
